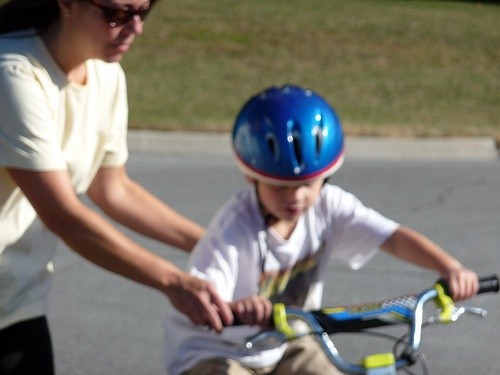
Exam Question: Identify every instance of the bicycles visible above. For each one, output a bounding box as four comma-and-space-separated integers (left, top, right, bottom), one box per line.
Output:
206, 274, 500, 375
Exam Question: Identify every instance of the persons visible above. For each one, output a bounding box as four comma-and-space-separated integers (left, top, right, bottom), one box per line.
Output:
165, 81, 479, 375
0, 0, 234, 375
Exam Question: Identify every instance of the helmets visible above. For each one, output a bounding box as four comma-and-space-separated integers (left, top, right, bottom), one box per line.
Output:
230, 84, 346, 186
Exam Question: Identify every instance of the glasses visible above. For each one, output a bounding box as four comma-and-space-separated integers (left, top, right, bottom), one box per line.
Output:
90, 0, 150, 27
